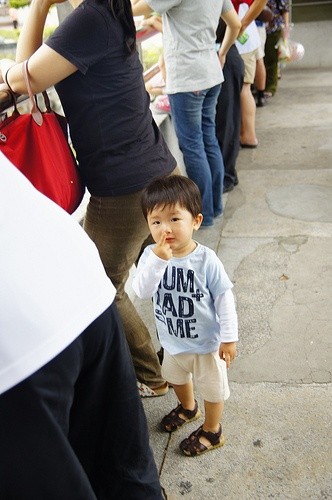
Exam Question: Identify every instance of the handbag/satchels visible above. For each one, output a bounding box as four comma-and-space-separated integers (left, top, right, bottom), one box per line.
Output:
0, 60, 85, 215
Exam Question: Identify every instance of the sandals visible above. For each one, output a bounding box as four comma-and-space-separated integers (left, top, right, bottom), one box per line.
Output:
180, 423, 225, 456
160, 399, 201, 433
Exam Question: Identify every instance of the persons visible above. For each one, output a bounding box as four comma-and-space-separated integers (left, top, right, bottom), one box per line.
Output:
131, 174, 239, 456
131, 0, 290, 227
0, 0, 182, 398
0, 148, 167, 500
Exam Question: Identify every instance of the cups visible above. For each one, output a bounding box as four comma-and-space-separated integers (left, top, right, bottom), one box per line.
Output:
239, 33, 249, 45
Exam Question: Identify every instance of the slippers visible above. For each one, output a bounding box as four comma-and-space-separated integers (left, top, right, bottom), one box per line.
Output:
136, 377, 169, 397
239, 142, 256, 148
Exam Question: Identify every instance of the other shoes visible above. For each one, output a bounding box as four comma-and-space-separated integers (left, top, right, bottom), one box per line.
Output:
256, 97, 266, 107
200, 225, 214, 229
217, 213, 223, 218
